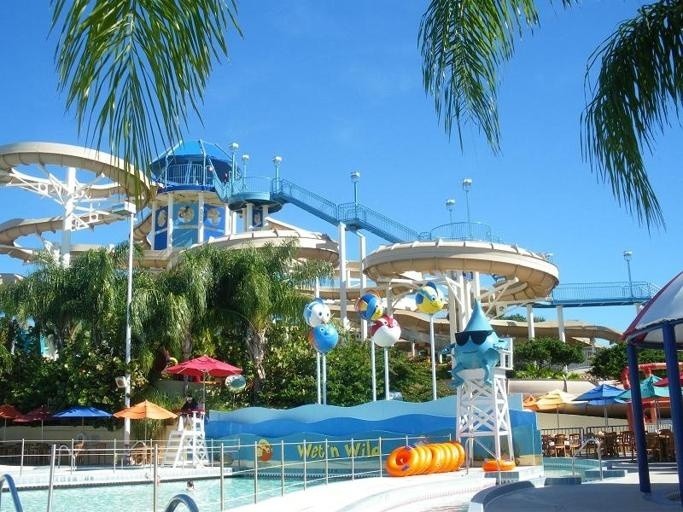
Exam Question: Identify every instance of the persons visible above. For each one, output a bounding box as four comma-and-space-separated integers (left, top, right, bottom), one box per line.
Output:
185, 479, 194, 491
181, 393, 199, 418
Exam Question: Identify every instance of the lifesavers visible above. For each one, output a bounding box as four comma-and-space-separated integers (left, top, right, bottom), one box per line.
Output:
483, 459, 516, 472
385, 441, 465, 477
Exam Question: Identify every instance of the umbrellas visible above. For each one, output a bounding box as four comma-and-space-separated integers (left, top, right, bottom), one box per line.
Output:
522, 374, 683, 433
166, 354, 242, 412
0, 399, 178, 454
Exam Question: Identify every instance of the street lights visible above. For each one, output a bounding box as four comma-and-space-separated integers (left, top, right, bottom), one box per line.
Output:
273, 156, 282, 192
229, 143, 239, 193
445, 197, 456, 237
624, 249, 633, 297
242, 154, 249, 192
463, 178, 473, 237
111, 202, 136, 465
351, 171, 360, 218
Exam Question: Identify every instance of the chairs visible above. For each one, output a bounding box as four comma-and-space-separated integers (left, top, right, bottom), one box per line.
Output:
0, 437, 105, 467
541, 429, 676, 462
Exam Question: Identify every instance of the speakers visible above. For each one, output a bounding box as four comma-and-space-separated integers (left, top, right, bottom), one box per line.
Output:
115, 376, 127, 388
